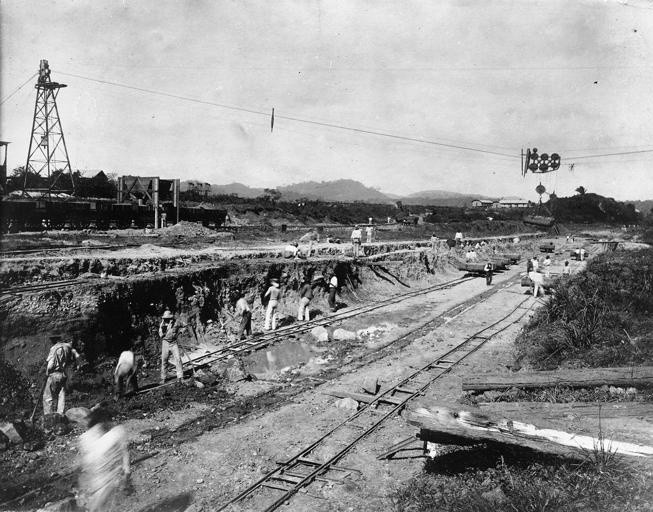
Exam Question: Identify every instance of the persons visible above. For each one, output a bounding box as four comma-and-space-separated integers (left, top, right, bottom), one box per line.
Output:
365, 225, 373, 243
351, 225, 362, 256
113, 351, 137, 398
293, 229, 319, 259
326, 271, 337, 310
65, 402, 131, 512
431, 234, 438, 248
527, 255, 571, 297
484, 260, 493, 285
158, 311, 188, 384
43, 334, 81, 416
232, 295, 252, 341
264, 282, 281, 329
455, 229, 462, 246
297, 279, 312, 321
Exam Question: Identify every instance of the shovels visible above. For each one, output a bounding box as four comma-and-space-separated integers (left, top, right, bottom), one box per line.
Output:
29, 375, 49, 429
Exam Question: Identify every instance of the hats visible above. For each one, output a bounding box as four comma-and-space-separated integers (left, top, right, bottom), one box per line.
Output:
162, 311, 173, 318
48, 328, 64, 339
